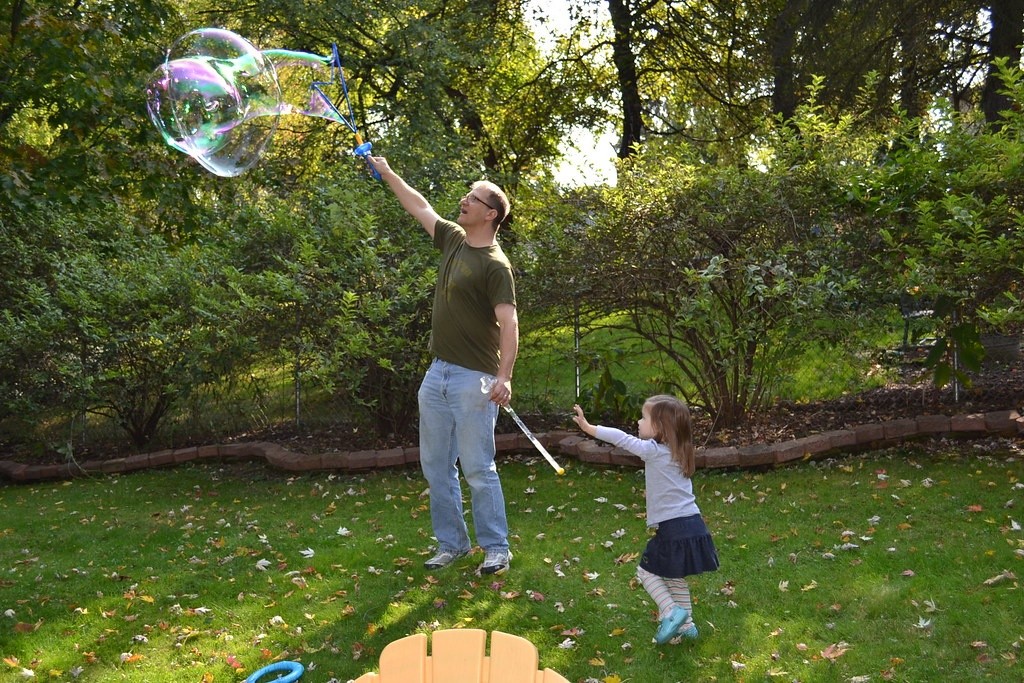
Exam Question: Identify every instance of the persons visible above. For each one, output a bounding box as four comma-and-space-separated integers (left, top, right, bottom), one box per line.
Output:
573, 396, 720, 644
368, 155, 519, 578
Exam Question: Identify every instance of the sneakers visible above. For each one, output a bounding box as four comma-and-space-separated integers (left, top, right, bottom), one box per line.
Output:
424, 547, 472, 572
480, 548, 513, 577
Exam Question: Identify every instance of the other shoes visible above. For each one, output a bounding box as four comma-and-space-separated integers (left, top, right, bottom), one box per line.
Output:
676, 625, 699, 639
657, 606, 688, 644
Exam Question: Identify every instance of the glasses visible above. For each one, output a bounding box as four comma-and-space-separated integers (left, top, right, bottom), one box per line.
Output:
460, 193, 499, 218
